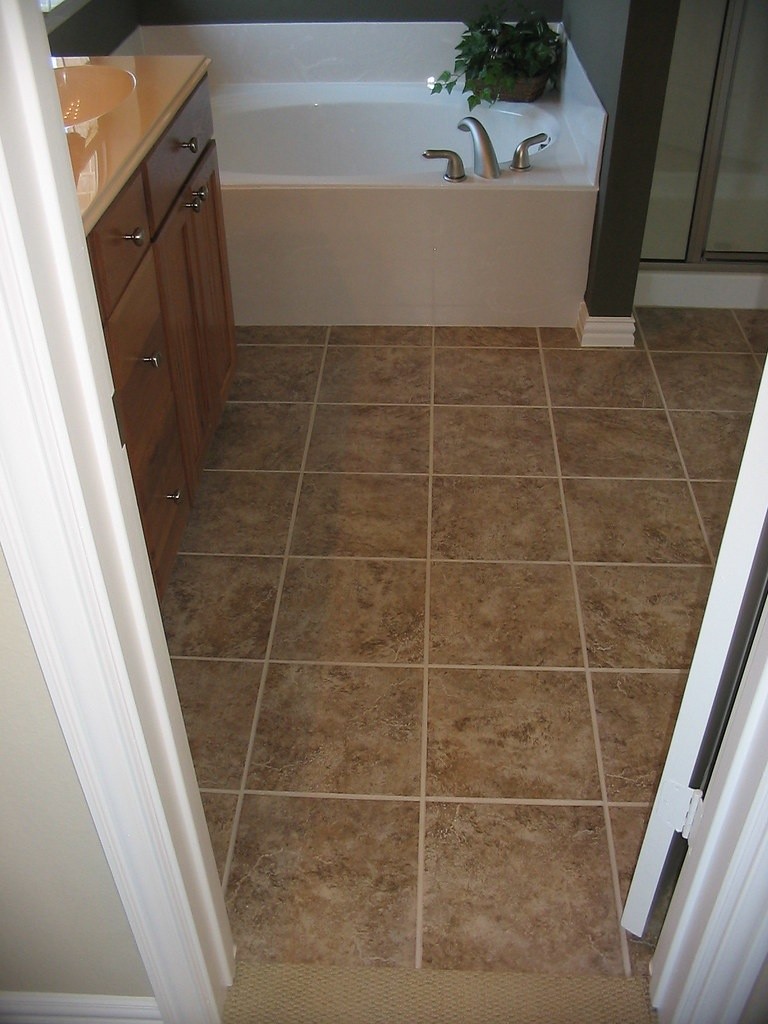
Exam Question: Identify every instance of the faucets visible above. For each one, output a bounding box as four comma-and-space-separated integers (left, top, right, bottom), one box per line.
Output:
457, 117, 500, 179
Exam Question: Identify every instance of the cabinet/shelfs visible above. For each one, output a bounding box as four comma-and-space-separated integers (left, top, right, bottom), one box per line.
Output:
50, 55, 237, 601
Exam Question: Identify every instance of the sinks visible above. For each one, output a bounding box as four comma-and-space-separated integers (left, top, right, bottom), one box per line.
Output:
54, 66, 136, 128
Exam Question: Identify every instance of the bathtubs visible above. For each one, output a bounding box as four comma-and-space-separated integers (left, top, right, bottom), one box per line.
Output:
107, 23, 608, 327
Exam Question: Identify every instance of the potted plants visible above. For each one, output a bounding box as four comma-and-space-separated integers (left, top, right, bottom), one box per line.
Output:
431, 10, 560, 111
